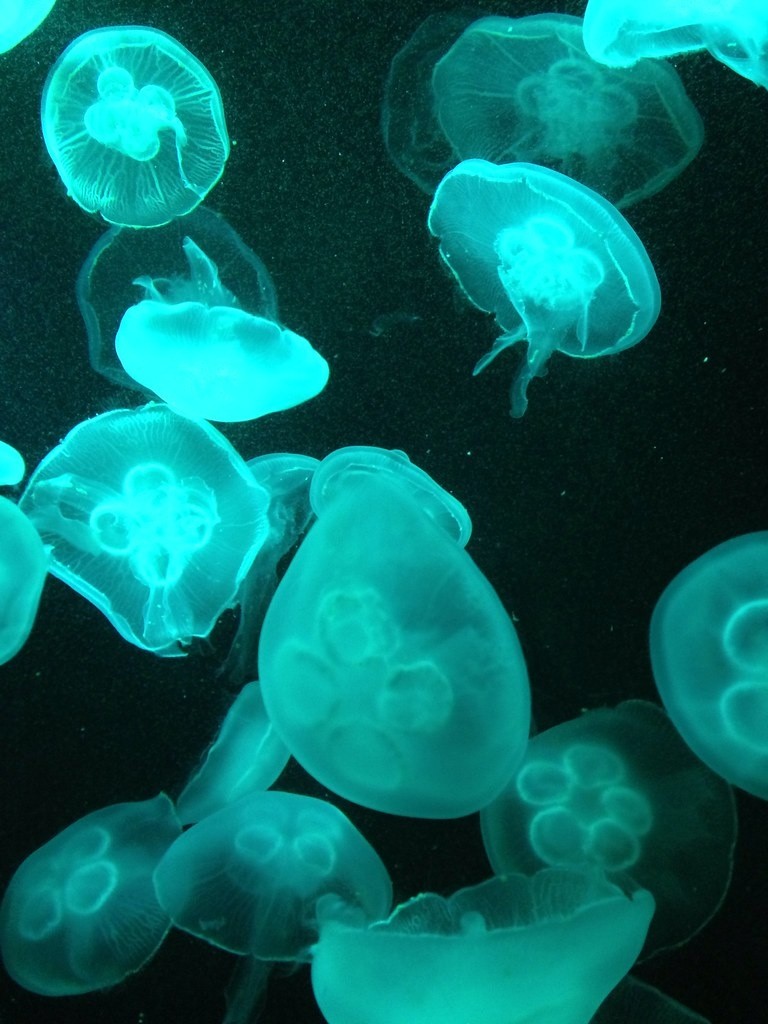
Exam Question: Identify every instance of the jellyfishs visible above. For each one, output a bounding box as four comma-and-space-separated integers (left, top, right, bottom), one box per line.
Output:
384, 0, 768, 421
0, 0, 335, 657
0, 444, 767, 1024
